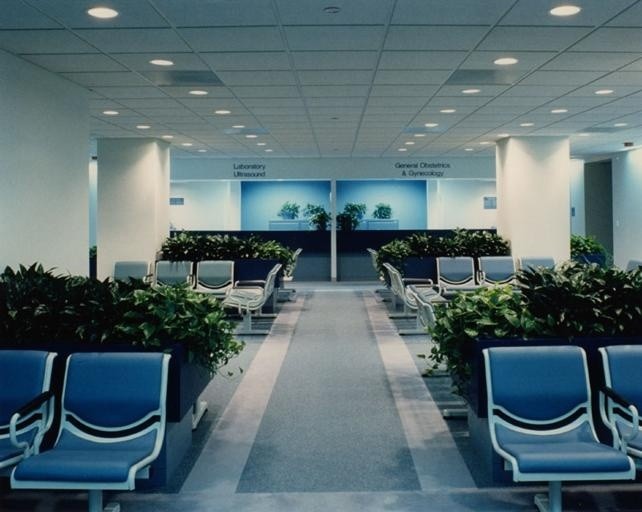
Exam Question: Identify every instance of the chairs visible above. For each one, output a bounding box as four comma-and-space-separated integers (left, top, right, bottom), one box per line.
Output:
599, 342, 642, 459
368, 248, 454, 378
436, 255, 556, 297
113, 259, 234, 299
7, 352, 172, 512
221, 248, 303, 337
0, 349, 57, 469
484, 344, 636, 512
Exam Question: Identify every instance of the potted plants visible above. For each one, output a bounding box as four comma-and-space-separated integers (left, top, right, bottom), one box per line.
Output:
276, 200, 391, 230
